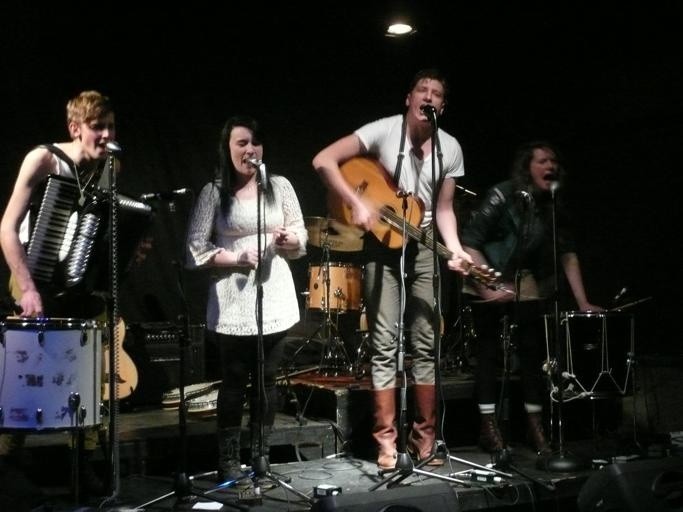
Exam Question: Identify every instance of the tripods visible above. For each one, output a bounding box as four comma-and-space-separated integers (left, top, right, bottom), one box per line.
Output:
366, 207, 472, 491
284, 242, 351, 378
439, 233, 557, 492
201, 195, 319, 506
387, 138, 513, 490
130, 226, 251, 512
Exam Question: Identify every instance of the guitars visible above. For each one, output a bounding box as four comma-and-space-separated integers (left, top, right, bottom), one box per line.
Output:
85, 290, 138, 416
326, 158, 503, 290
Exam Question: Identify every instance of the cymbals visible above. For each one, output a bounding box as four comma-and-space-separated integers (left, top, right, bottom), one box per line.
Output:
303, 216, 364, 252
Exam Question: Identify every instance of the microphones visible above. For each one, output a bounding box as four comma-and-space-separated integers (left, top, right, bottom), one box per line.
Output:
422, 104, 443, 157
548, 180, 560, 197
102, 140, 121, 152
246, 158, 269, 193
142, 186, 197, 206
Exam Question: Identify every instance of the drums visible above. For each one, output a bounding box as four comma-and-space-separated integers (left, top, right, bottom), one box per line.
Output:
308, 262, 362, 310
559, 308, 636, 398
0, 317, 102, 432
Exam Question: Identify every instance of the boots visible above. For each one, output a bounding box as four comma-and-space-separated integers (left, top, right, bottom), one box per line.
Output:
408, 383, 444, 467
478, 412, 505, 453
215, 424, 253, 489
369, 386, 400, 469
526, 413, 551, 452
249, 421, 291, 484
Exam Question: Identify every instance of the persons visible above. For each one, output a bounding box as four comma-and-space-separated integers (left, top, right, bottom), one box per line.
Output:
1, 89, 122, 499
185, 116, 309, 490
312, 68, 474, 475
457, 143, 608, 454
